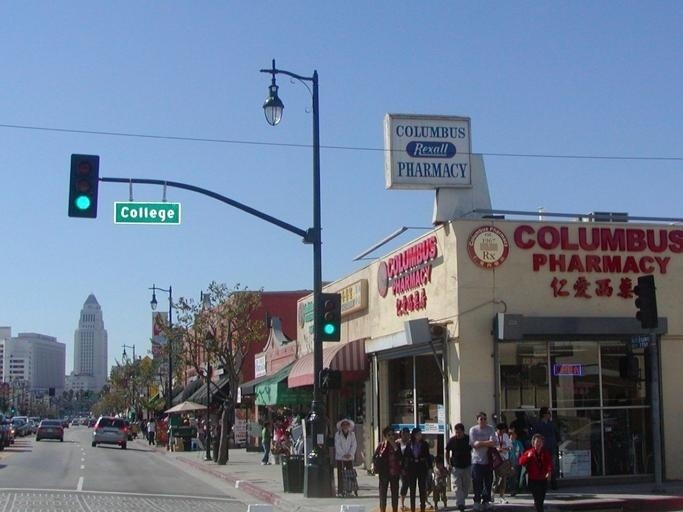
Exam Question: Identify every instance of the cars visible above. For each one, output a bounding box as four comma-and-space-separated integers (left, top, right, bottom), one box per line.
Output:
125, 421, 132, 440
0, 412, 98, 454
568, 416, 616, 438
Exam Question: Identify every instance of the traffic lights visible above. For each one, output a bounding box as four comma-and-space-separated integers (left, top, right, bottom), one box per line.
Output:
67, 152, 100, 219
319, 291, 341, 343
320, 368, 340, 391
632, 274, 658, 331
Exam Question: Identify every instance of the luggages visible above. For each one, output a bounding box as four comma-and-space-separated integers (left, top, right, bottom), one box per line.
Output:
340, 455, 357, 498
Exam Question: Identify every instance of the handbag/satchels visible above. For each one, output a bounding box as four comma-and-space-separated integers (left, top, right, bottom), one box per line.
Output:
487, 447, 501, 470
494, 460, 510, 477
518, 466, 527, 489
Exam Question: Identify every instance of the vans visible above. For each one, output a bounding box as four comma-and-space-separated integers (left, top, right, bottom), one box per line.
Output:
90, 414, 130, 451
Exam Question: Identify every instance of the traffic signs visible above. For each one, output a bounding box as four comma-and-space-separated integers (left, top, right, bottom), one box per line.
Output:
115, 200, 183, 225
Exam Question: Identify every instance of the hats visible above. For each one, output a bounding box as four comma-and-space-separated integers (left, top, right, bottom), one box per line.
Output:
336, 418, 354, 431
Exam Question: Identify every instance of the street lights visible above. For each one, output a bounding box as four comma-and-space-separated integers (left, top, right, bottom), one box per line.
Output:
147, 282, 175, 412
121, 343, 138, 403
258, 58, 338, 500
203, 328, 216, 463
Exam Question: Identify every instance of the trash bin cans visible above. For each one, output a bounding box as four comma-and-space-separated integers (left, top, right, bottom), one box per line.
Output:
281, 454, 304, 493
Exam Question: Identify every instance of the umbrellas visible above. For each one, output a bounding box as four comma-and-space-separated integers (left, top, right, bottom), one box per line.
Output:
164, 401, 211, 419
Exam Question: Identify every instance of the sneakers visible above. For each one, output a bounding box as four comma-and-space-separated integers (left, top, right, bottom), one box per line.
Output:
473, 502, 480, 510
481, 502, 490, 510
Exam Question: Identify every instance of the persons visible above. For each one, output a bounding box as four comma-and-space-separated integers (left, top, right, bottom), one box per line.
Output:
371, 407, 557, 512
140, 418, 155, 445
335, 419, 358, 497
261, 421, 273, 465
